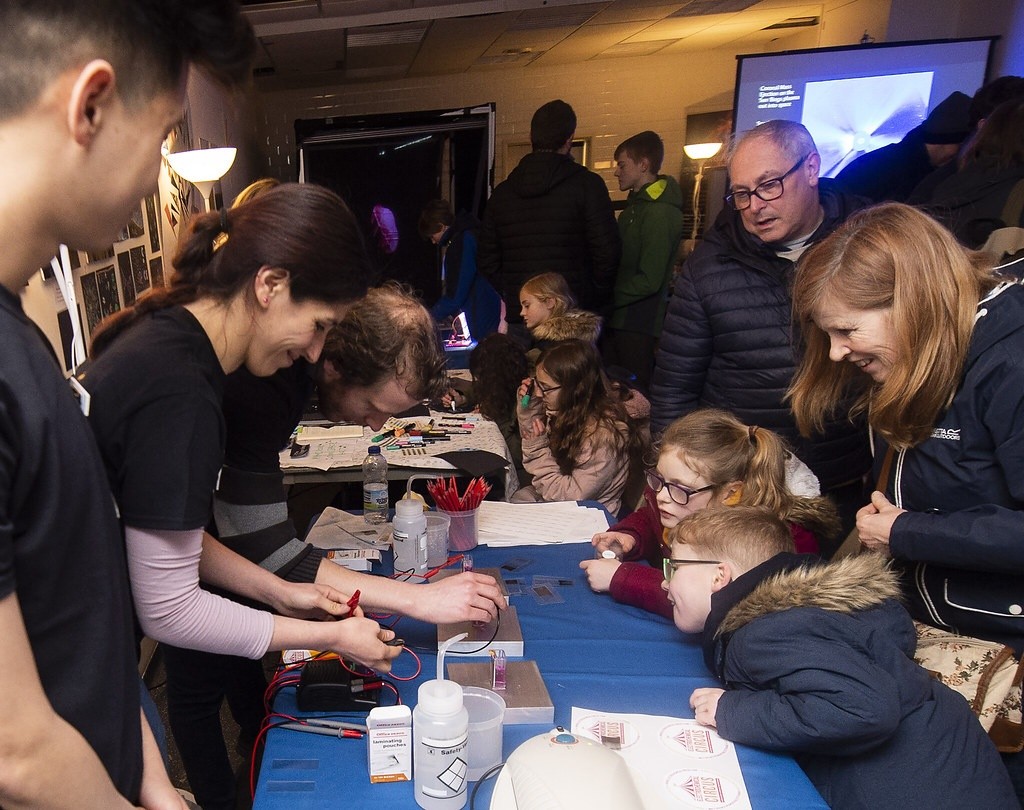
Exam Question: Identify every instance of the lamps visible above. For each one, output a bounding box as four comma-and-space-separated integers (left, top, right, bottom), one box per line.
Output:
684, 142, 725, 254
161, 146, 239, 214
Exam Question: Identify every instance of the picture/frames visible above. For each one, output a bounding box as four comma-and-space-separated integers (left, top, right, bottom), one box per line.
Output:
500, 135, 592, 187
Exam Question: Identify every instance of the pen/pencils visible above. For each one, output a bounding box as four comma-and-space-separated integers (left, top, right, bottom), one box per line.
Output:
438, 424, 474, 428
395, 441, 435, 446
429, 431, 471, 434
442, 417, 479, 421
426, 475, 493, 551
386, 443, 426, 450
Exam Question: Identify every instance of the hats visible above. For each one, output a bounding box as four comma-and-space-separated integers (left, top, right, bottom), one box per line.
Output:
922, 89, 976, 145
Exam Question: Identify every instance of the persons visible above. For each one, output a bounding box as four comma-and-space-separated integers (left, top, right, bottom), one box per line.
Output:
644, 111, 895, 522
601, 129, 686, 386
446, 330, 536, 500
783, 197, 1024, 657
1, 0, 258, 810
834, 70, 1024, 280
661, 504, 1024, 810
74, 173, 509, 810
477, 98, 622, 358
517, 271, 603, 377
513, 333, 652, 520
582, 402, 839, 618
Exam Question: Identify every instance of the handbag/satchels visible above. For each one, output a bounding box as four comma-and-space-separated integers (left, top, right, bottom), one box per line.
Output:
913, 620, 1024, 754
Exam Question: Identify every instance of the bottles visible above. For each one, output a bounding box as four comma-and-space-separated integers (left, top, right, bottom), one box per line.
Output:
362, 446, 389, 525
413, 679, 469, 810
393, 499, 428, 585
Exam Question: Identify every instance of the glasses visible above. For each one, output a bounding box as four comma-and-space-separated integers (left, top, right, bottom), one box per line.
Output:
722, 153, 807, 211
534, 376, 563, 397
664, 558, 734, 586
644, 463, 729, 505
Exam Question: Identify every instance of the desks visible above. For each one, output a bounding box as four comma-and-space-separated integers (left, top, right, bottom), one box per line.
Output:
247, 496, 833, 810
281, 369, 520, 501
443, 336, 478, 368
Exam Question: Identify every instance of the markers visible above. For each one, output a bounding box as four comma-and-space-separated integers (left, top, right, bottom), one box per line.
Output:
409, 436, 450, 441
521, 376, 537, 408
372, 429, 395, 442
394, 423, 416, 437
446, 376, 456, 411
426, 419, 435, 431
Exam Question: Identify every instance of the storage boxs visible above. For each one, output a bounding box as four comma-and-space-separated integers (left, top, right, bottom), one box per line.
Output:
364, 705, 413, 784
327, 549, 383, 572
281, 649, 338, 669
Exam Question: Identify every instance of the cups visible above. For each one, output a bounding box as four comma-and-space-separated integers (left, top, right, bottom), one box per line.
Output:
436, 498, 480, 551
462, 686, 506, 782
423, 512, 451, 567
